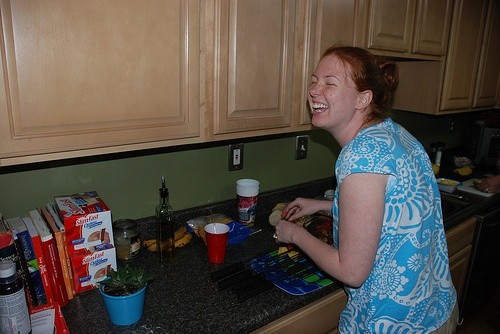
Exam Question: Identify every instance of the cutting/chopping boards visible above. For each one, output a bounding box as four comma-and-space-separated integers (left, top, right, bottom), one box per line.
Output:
457, 178, 496, 197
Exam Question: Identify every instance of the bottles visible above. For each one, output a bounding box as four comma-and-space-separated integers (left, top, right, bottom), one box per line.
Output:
155, 188, 175, 263
0, 261, 32, 334
113, 218, 141, 259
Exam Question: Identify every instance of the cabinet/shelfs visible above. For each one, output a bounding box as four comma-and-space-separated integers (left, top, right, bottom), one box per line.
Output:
0, 0, 500, 167
53, 174, 477, 334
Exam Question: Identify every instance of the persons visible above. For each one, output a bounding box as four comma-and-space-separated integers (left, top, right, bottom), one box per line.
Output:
274, 46, 460, 334
473, 174, 500, 193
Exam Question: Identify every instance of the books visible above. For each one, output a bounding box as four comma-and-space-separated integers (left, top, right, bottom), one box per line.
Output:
6, 203, 76, 307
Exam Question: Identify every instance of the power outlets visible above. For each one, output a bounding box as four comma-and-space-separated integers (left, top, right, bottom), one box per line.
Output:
228, 143, 244, 171
295, 136, 308, 159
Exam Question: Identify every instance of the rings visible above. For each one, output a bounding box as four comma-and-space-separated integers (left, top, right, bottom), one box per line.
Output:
273, 234, 277, 239
486, 188, 488, 192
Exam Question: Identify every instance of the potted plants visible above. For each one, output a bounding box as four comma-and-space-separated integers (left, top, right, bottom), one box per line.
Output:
95, 264, 156, 326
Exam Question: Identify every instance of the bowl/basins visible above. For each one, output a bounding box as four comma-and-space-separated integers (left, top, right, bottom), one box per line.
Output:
436, 178, 461, 193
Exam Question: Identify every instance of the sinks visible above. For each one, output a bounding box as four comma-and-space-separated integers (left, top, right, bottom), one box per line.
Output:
438, 190, 477, 226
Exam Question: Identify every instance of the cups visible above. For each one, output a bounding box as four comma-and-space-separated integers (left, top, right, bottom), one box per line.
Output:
236, 179, 260, 225
204, 223, 230, 263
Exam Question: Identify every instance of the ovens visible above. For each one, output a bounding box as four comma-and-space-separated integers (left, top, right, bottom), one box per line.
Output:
464, 120, 500, 165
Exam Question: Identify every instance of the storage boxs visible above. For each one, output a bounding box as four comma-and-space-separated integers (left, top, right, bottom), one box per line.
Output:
54, 190, 115, 257
71, 247, 118, 293
436, 178, 464, 193
